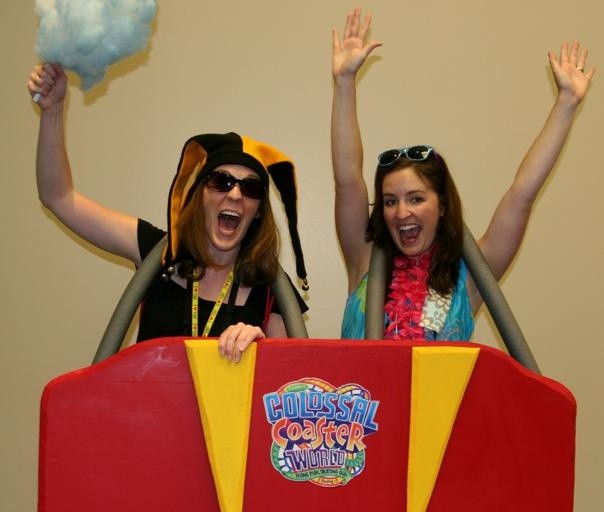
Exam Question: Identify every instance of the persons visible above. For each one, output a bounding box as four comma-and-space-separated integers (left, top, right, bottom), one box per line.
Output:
25, 60, 311, 365
331, 4, 597, 343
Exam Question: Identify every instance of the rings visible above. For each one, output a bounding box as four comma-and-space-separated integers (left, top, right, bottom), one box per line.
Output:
575, 66, 585, 72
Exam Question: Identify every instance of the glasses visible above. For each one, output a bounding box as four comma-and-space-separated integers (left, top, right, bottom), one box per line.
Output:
376, 144, 445, 172
205, 171, 264, 200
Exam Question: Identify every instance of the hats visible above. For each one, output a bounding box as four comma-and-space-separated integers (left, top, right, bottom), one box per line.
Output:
154, 130, 313, 291
